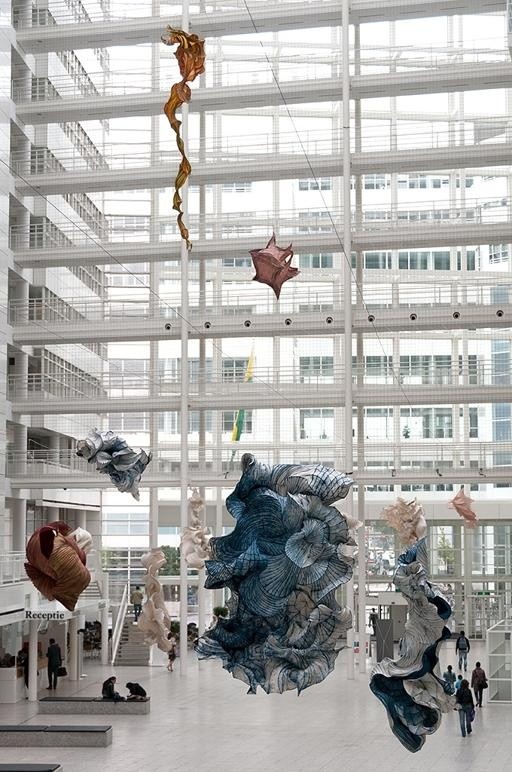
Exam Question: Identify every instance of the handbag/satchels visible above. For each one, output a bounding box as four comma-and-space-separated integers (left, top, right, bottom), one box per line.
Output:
57, 667, 68, 676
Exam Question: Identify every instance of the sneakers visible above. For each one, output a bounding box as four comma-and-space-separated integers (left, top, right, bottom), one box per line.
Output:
45, 686, 57, 690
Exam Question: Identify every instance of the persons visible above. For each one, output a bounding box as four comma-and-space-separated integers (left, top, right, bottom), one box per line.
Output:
102, 676, 117, 697
470, 661, 488, 706
368, 607, 378, 635
46, 638, 63, 690
455, 678, 474, 737
443, 665, 456, 698
454, 674, 463, 691
455, 630, 470, 671
126, 682, 147, 700
84, 631, 102, 651
17, 647, 30, 699
130, 585, 143, 622
166, 644, 176, 672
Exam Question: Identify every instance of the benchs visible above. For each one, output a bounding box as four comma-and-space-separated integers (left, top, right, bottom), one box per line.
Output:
0, 764, 64, 772
0, 724, 114, 748
36, 695, 150, 715
